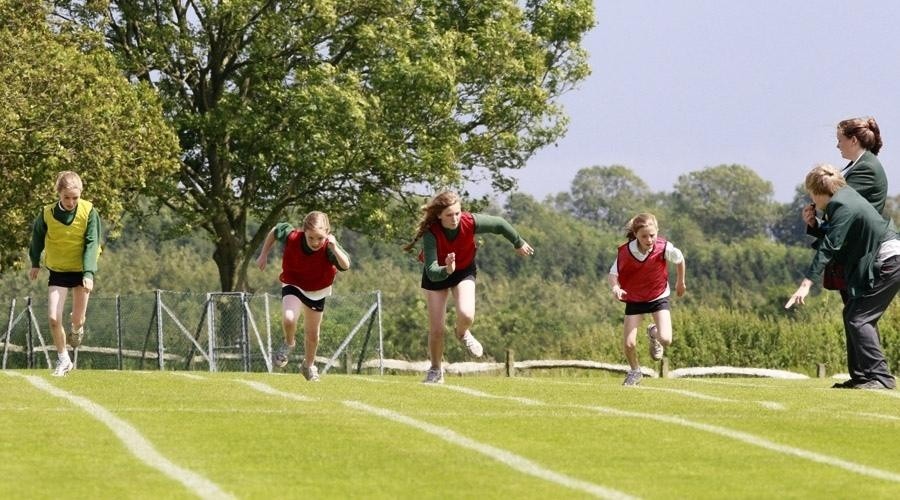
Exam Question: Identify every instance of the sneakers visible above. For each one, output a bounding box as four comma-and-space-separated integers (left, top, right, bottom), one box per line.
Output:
455, 325, 486, 359
275, 338, 296, 367
69, 312, 84, 346
53, 358, 73, 377
300, 359, 320, 383
855, 378, 894, 392
419, 366, 444, 387
647, 324, 666, 362
623, 366, 642, 388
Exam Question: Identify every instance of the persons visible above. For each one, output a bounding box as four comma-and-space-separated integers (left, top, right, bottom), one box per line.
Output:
608, 211, 686, 388
834, 116, 888, 388
784, 164, 900, 390
28, 171, 101, 377
403, 193, 535, 382
256, 210, 351, 381
